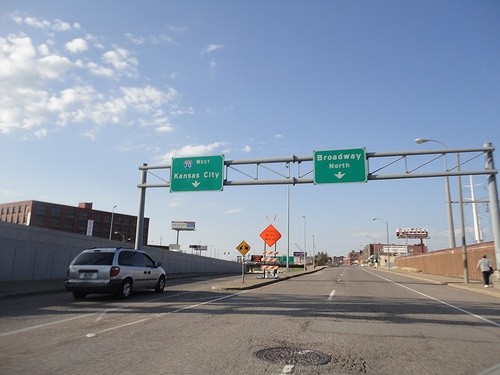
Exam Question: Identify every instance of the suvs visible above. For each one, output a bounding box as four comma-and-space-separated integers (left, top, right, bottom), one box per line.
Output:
64, 246, 166, 300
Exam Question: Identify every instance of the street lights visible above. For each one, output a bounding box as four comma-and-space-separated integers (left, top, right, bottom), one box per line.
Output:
414, 137, 469, 284
109, 205, 117, 240
302, 215, 306, 271
311, 235, 314, 269
115, 232, 132, 242
365, 236, 375, 266
198, 240, 201, 255
372, 217, 390, 270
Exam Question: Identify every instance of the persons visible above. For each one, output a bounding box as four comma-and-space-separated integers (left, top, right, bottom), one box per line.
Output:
477, 255, 492, 288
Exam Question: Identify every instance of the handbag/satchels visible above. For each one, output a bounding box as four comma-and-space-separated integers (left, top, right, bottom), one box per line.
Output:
488, 266, 494, 275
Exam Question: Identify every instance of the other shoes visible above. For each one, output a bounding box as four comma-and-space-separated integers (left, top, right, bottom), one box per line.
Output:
484, 284, 490, 288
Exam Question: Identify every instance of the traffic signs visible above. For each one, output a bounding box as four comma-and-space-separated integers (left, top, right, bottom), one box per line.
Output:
312, 147, 368, 185
168, 154, 224, 193
